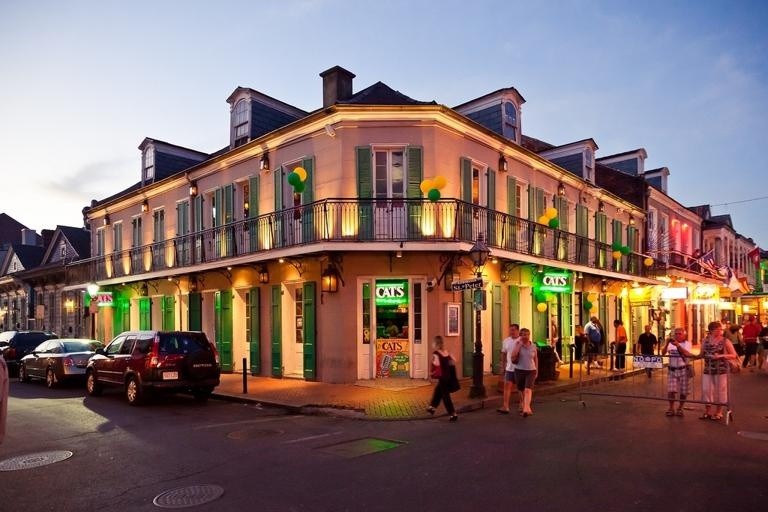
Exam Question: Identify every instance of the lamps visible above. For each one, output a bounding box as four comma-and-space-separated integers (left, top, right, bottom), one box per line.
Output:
137, 260, 346, 297
436, 256, 462, 293
496, 154, 636, 228
95, 150, 273, 227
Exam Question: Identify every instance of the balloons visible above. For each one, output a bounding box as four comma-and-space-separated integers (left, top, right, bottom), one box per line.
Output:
589, 306, 599, 314
621, 245, 632, 257
293, 182, 307, 194
584, 301, 593, 310
547, 293, 557, 305
611, 251, 623, 260
535, 292, 547, 302
293, 167, 309, 182
612, 240, 623, 251
287, 170, 302, 186
536, 303, 549, 314
539, 215, 550, 226
433, 175, 448, 189
586, 293, 598, 303
645, 257, 655, 267
546, 207, 559, 219
427, 188, 440, 202
419, 178, 434, 195
549, 218, 561, 230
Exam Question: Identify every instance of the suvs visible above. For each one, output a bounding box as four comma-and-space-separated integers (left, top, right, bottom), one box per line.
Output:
83, 330, 223, 410
0, 328, 61, 379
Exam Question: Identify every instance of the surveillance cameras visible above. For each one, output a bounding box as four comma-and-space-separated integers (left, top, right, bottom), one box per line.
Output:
426, 278, 437, 287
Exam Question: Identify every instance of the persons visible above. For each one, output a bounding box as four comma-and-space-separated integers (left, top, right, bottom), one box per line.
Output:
510, 327, 539, 417
660, 326, 695, 418
424, 335, 459, 423
551, 313, 564, 373
595, 320, 606, 358
496, 323, 524, 413
385, 320, 400, 337
582, 316, 603, 369
689, 320, 739, 422
613, 320, 628, 371
725, 316, 767, 373
636, 324, 659, 379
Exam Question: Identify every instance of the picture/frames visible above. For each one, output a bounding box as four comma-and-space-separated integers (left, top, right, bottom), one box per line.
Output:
445, 302, 462, 339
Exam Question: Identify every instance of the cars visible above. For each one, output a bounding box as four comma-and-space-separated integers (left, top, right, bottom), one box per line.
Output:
14, 337, 109, 391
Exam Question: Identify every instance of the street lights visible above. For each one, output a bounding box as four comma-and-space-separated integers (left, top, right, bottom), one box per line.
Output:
467, 231, 488, 401
86, 281, 98, 341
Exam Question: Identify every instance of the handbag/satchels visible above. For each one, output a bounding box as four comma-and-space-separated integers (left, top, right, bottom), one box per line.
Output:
723, 347, 742, 372
512, 352, 519, 364
430, 365, 442, 378
686, 366, 695, 377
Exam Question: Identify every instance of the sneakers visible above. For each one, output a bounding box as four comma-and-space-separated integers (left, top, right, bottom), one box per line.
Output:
497, 407, 509, 413
449, 414, 457, 421
699, 412, 723, 420
426, 407, 435, 414
518, 403, 533, 417
665, 408, 683, 416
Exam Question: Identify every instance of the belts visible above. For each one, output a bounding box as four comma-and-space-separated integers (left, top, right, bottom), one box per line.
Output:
668, 366, 685, 370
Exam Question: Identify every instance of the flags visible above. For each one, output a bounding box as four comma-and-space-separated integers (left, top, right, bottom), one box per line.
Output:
696, 253, 752, 296
747, 247, 761, 271
